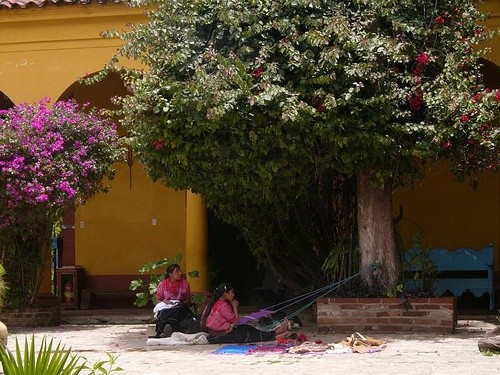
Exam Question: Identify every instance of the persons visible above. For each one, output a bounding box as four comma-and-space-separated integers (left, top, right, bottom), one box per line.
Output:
202, 282, 290, 343
154, 263, 193, 337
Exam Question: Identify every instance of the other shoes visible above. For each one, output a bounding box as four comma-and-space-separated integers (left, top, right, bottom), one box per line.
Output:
160, 324, 172, 337
283, 317, 288, 333
276, 331, 292, 338
